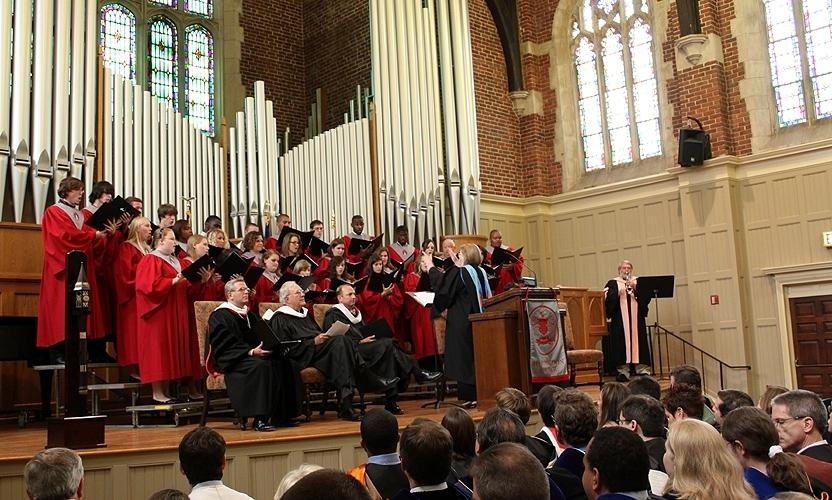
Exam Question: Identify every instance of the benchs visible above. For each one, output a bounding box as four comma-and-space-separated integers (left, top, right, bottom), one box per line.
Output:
32, 350, 462, 429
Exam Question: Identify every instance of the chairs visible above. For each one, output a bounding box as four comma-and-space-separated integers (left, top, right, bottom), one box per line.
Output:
190, 299, 388, 429
556, 301, 605, 388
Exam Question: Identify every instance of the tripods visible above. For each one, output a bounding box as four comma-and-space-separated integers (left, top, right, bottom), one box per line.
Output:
422, 307, 467, 411
654, 289, 671, 381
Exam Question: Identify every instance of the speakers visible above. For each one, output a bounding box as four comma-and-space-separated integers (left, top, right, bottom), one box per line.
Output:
678, 129, 705, 166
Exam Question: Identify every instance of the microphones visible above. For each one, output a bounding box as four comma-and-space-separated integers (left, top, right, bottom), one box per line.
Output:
504, 251, 536, 287
626, 272, 631, 291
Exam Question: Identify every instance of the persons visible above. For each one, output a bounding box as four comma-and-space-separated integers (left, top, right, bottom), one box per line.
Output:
36, 177, 524, 430
24, 448, 84, 500
603, 260, 651, 381
149, 365, 832, 500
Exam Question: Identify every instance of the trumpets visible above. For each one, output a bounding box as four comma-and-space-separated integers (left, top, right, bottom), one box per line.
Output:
624, 272, 634, 295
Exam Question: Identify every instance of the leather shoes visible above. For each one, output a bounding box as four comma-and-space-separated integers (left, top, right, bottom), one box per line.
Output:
153, 398, 176, 404
374, 375, 398, 394
253, 419, 274, 431
339, 408, 362, 421
415, 370, 442, 384
282, 418, 300, 426
384, 403, 404, 414
